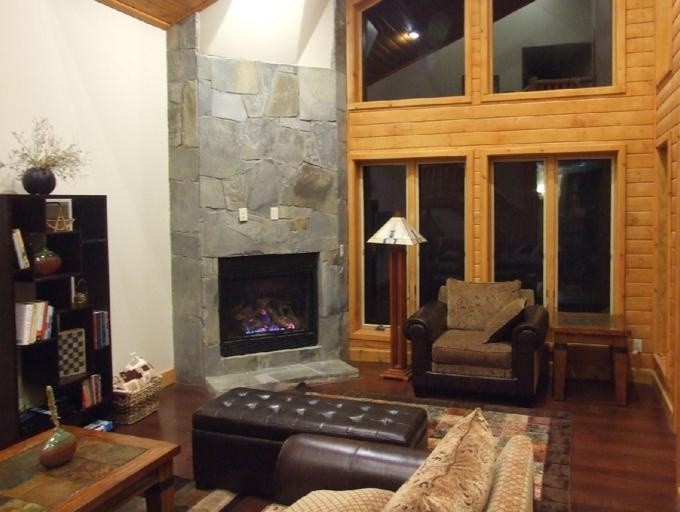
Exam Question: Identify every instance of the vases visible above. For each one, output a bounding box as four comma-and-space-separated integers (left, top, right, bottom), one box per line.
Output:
38, 428, 78, 468
22, 168, 56, 194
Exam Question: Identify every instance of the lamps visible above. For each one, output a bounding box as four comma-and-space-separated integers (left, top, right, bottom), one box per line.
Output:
365, 208, 428, 382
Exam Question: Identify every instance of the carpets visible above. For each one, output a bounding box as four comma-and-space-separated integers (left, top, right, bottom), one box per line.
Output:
116, 474, 274, 511
300, 388, 572, 512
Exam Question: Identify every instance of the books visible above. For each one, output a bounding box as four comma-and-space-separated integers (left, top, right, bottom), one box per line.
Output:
15, 299, 54, 346
78, 374, 103, 409
83, 309, 110, 349
11, 227, 31, 270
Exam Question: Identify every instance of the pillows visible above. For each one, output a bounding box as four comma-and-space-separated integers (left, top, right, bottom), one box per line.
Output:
281, 407, 498, 512
445, 276, 528, 344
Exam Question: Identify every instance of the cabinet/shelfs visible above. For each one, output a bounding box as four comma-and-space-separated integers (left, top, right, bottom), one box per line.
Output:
0, 193, 114, 450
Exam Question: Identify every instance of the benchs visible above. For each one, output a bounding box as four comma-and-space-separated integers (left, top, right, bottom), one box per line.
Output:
191, 386, 428, 499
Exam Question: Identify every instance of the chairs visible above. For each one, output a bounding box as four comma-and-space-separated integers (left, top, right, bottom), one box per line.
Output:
403, 285, 549, 409
258, 434, 535, 512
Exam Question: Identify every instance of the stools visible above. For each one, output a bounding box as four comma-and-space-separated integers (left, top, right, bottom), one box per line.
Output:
549, 311, 631, 406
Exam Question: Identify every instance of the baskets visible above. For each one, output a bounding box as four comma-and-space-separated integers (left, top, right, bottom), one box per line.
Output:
113, 381, 160, 425
